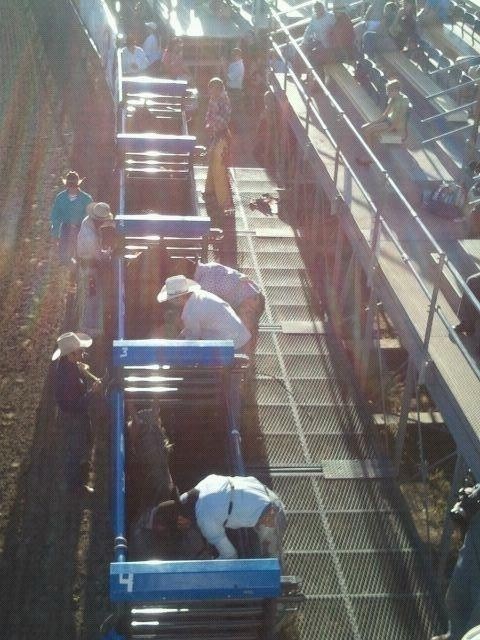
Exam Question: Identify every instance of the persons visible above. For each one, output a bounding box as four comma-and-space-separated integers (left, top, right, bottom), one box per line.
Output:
354, 79, 410, 167
450, 270, 479, 333
173, 255, 266, 355
159, 36, 193, 83
133, 21, 163, 68
155, 273, 252, 356
153, 472, 290, 559
118, 33, 151, 76
284, 0, 452, 91
49, 329, 105, 499
76, 202, 118, 336
201, 77, 241, 206
223, 47, 245, 134
50, 170, 93, 267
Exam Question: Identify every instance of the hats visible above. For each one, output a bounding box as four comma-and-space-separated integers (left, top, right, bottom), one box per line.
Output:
62, 170, 88, 187
154, 486, 182, 547
85, 201, 115, 222
51, 331, 94, 362
144, 22, 158, 30
180, 256, 199, 280
156, 274, 203, 304
